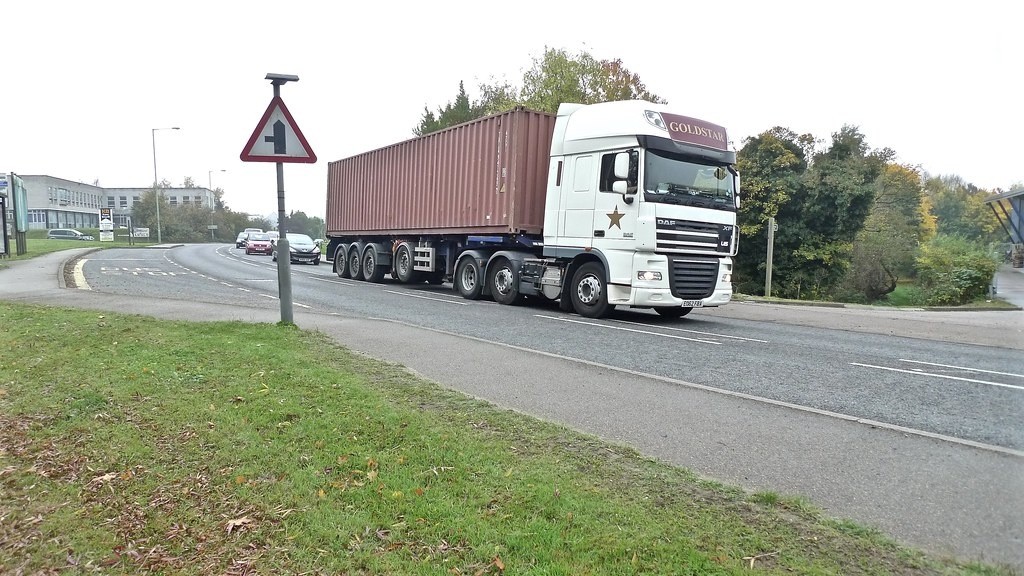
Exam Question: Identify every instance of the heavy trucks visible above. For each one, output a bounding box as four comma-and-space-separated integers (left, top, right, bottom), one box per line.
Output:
324, 100, 741, 319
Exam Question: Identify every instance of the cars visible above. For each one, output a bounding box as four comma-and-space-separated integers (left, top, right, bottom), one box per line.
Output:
266, 231, 280, 239
246, 233, 271, 255
271, 234, 321, 265
236, 231, 249, 248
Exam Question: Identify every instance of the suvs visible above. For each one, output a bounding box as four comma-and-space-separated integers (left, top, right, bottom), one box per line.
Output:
47, 229, 95, 241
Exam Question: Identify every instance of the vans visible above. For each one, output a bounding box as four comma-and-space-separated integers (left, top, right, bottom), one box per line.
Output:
245, 228, 262, 234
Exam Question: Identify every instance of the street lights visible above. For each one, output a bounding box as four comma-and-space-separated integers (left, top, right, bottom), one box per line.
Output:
152, 127, 180, 244
209, 170, 226, 238
